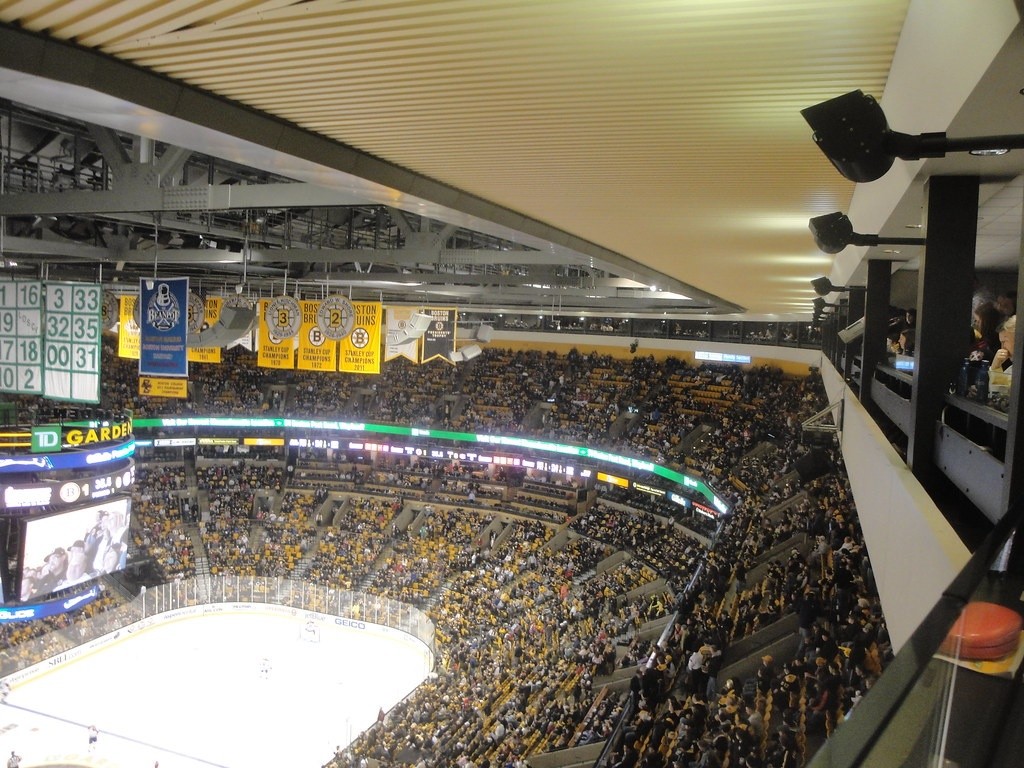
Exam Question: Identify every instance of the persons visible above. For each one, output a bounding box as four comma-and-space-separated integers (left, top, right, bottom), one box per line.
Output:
0, 287, 1023, 768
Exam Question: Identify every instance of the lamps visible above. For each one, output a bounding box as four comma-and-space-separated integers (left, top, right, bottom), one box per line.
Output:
807, 212, 925, 253
807, 275, 866, 333
801, 88, 1024, 185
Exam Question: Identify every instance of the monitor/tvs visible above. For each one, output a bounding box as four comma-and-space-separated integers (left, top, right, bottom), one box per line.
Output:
17, 494, 133, 602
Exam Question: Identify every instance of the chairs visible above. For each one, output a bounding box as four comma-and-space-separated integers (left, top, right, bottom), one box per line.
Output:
1, 340, 895, 767
